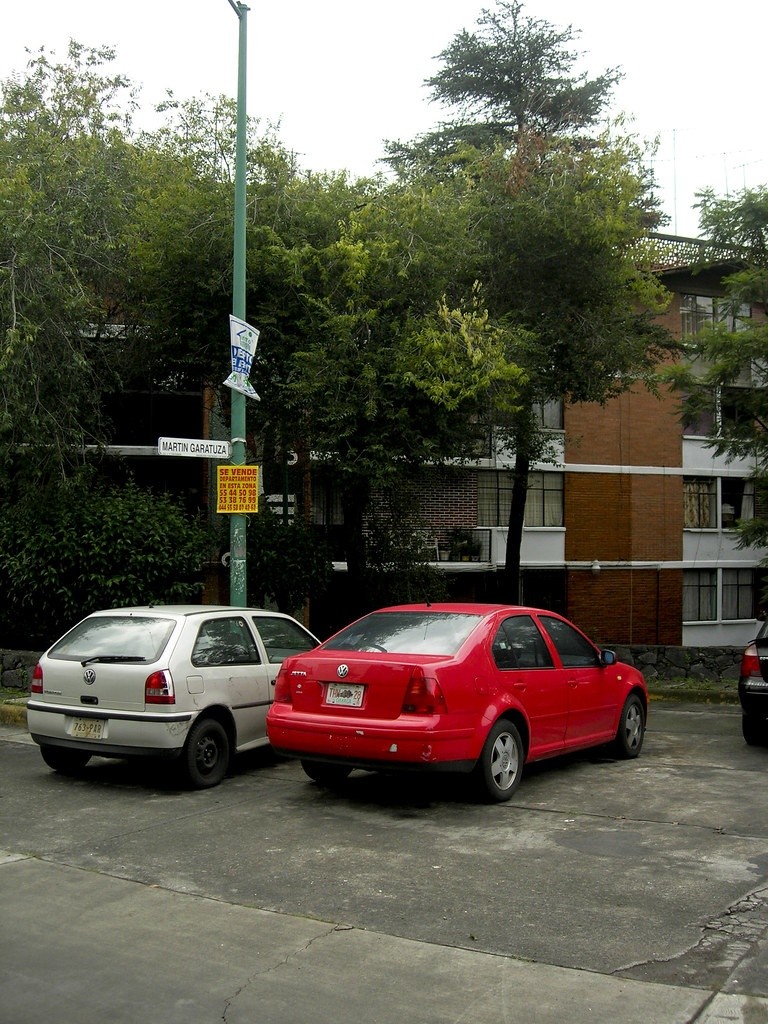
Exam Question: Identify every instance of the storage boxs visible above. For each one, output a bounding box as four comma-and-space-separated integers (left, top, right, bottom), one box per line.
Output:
722, 503, 734, 521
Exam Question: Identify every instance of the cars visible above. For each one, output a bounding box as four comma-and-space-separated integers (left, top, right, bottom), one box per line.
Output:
265, 601, 650, 803
736, 606, 768, 744
24, 605, 327, 790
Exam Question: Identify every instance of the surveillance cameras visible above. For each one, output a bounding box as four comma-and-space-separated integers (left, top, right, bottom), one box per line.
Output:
591, 560, 601, 575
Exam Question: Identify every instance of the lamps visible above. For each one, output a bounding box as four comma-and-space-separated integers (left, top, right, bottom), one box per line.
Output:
591, 560, 601, 576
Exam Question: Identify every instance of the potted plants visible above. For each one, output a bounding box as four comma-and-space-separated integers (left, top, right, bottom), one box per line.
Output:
438, 527, 482, 562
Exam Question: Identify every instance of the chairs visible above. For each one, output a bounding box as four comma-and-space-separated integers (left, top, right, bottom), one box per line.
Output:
387, 530, 440, 564
